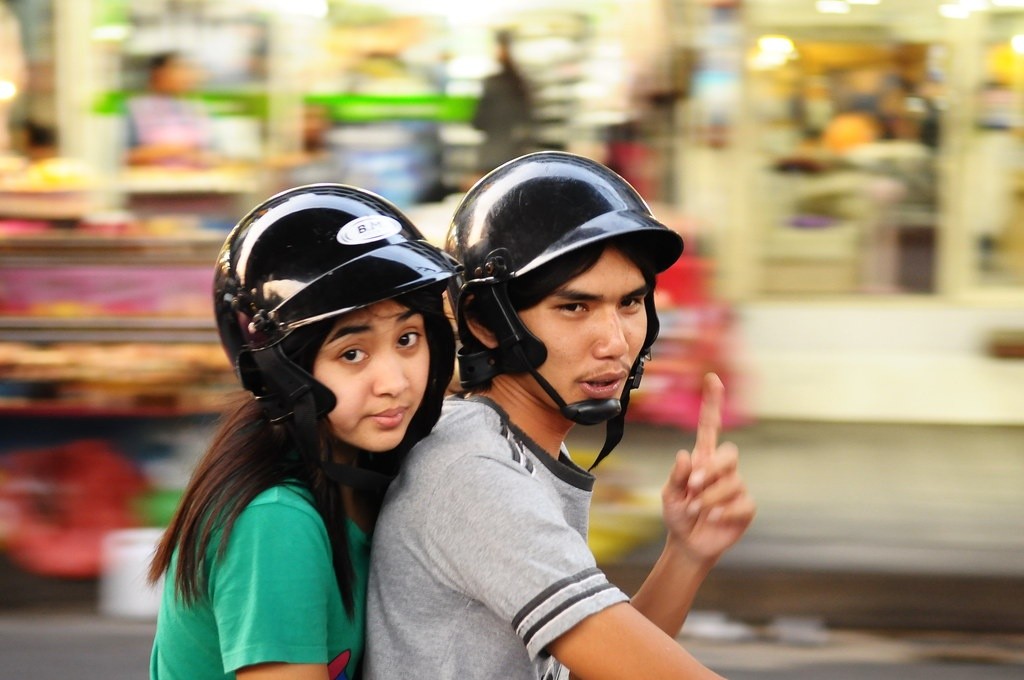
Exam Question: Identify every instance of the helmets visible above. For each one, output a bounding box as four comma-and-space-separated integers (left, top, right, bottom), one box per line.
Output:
211, 179, 460, 485
443, 150, 685, 472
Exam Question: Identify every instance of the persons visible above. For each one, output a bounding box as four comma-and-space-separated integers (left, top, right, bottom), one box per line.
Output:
470, 28, 542, 175
362, 149, 756, 680
148, 182, 463, 680
125, 48, 240, 229
